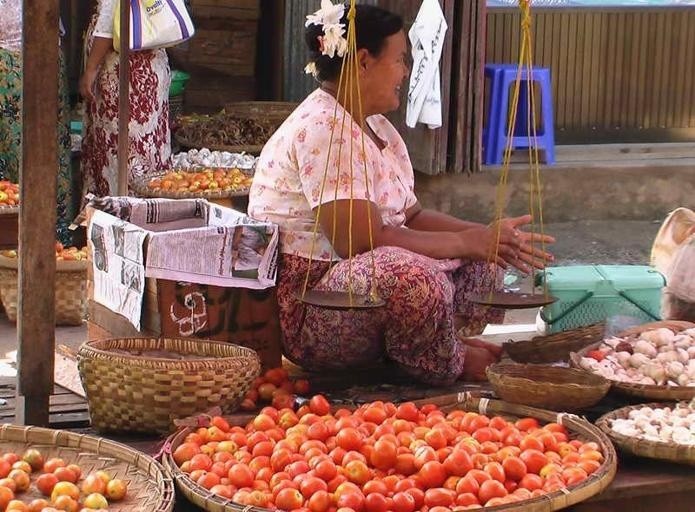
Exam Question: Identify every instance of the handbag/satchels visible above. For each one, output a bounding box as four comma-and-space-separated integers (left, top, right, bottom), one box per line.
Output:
112, 0, 196, 53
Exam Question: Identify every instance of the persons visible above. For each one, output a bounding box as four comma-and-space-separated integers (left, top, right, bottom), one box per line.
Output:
1, 1, 77, 250
246, 0, 558, 389
71, 0, 172, 250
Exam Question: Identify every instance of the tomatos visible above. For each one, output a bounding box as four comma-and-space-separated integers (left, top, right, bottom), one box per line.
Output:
0, 180, 20, 207
148, 168, 254, 193
173, 367, 603, 512
2, 240, 88, 262
0, 448, 126, 512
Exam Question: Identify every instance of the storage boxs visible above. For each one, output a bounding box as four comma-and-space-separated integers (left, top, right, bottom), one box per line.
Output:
84, 198, 283, 374
539, 263, 666, 337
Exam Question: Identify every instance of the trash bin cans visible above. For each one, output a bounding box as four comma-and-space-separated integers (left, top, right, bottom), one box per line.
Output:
71, 134, 83, 245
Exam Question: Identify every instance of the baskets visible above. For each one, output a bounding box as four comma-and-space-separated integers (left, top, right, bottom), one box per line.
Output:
0, 254, 88, 326
162, 396, 617, 510
1, 205, 19, 215
76, 335, 262, 436
224, 99, 299, 124
1, 424, 175, 510
131, 167, 255, 199
176, 133, 263, 159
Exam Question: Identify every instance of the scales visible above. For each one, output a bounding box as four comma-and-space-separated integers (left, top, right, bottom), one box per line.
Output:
297, 0, 560, 310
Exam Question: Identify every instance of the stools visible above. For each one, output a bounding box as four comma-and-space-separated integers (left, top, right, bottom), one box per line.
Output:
481, 63, 556, 167
